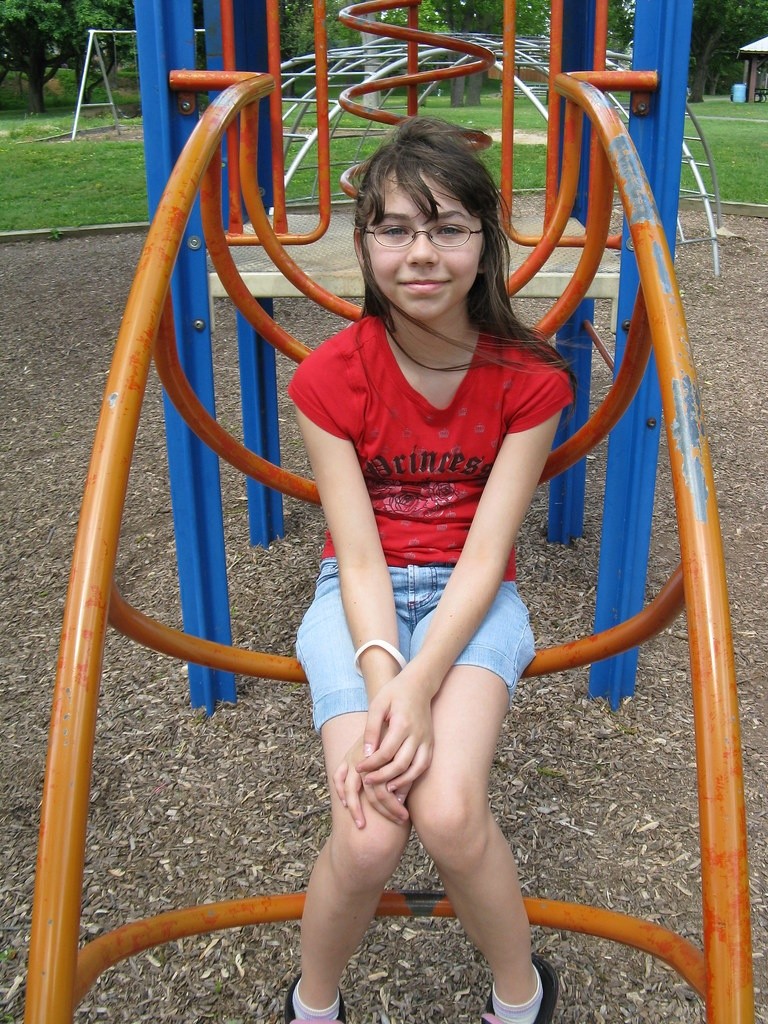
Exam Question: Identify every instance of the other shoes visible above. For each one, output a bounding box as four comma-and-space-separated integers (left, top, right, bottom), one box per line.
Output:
481, 955, 558, 1024
285, 973, 346, 1024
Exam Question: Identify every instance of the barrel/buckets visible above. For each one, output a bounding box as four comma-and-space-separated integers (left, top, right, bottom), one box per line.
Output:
732, 82, 746, 103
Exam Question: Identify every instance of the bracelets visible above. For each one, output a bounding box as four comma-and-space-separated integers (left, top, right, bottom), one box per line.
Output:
353, 638, 407, 678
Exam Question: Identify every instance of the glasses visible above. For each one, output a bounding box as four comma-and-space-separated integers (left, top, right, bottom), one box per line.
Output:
363, 223, 484, 249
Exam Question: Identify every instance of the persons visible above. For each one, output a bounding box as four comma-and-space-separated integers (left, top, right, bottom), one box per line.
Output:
282, 117, 579, 1024
